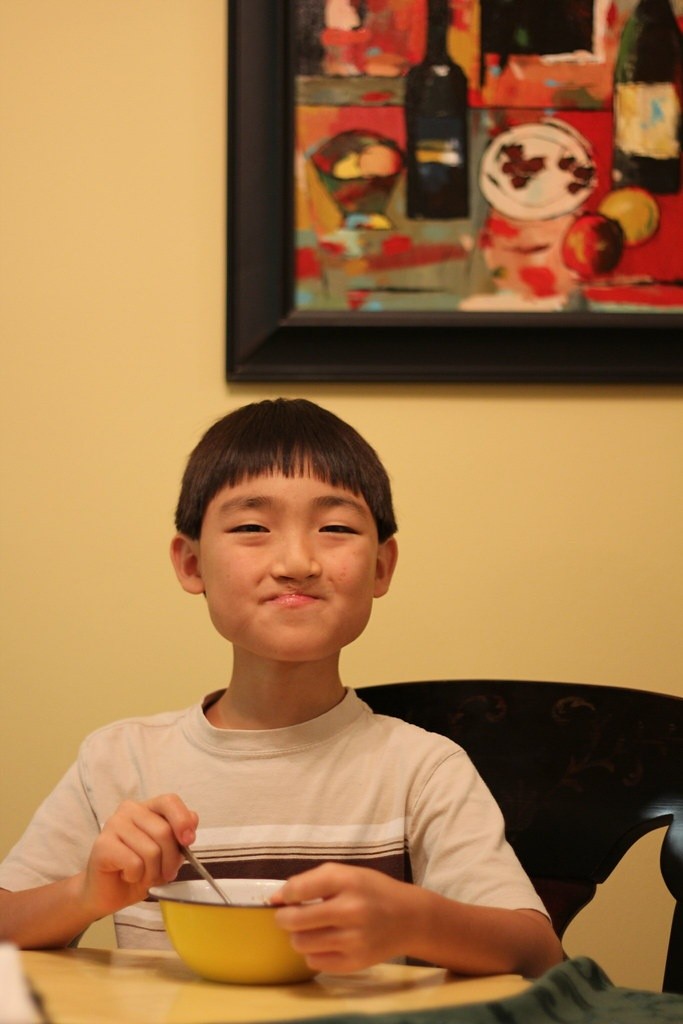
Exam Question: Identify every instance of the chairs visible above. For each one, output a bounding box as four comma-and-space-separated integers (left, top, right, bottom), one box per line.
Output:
350, 679, 682, 996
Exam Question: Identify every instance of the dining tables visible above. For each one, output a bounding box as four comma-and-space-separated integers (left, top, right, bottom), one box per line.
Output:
0, 946, 531, 1024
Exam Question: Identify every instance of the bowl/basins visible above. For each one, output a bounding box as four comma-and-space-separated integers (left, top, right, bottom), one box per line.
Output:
147, 879, 323, 984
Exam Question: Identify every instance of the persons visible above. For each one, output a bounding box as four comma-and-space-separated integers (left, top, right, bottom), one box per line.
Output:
0, 398, 563, 978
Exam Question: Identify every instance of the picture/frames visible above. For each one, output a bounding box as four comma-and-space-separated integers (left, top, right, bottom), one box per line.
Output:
225, 0, 682, 387
